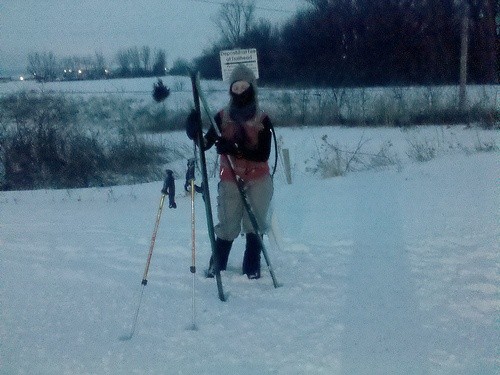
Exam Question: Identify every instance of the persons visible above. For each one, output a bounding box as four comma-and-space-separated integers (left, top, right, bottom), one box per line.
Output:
185, 64, 275, 280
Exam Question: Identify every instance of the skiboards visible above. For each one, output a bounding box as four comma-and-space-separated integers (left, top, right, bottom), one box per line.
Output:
185, 67, 285, 302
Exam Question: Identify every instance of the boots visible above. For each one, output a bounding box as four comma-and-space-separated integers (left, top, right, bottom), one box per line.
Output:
207, 236, 235, 278
243, 232, 264, 279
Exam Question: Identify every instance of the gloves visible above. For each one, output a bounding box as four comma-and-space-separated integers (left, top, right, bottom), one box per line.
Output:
217, 140, 248, 158
187, 108, 202, 139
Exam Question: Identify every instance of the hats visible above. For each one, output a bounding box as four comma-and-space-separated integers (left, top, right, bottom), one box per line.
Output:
228, 62, 260, 98
